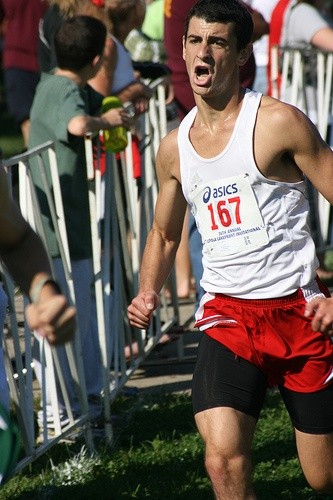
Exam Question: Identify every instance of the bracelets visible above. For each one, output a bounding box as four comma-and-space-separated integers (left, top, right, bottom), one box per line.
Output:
29, 278, 63, 303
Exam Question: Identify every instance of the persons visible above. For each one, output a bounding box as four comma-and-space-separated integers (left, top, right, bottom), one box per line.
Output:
1, 12, 332, 397
0, 183, 78, 347
125, 0, 333, 500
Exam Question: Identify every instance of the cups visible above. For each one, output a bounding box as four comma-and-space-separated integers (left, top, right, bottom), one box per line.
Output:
102, 96, 128, 153
